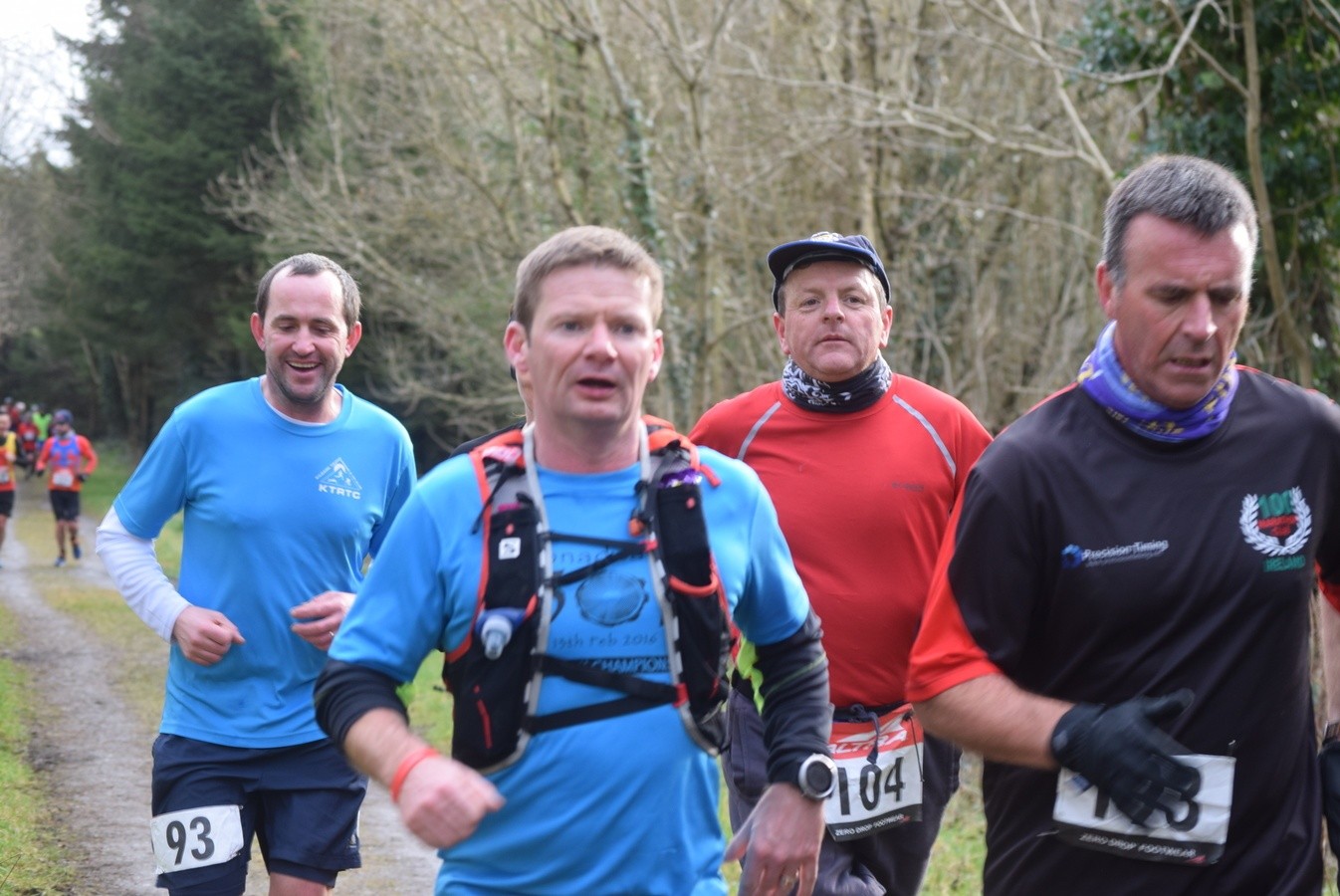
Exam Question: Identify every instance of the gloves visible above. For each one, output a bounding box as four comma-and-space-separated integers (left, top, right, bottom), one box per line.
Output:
79, 473, 88, 482
1313, 722, 1340, 863
1052, 687, 1192, 829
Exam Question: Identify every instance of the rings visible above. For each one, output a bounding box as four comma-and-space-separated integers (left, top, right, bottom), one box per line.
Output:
780, 875, 797, 888
328, 632, 335, 641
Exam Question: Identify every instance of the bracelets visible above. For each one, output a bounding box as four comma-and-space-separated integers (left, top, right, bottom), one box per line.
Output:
389, 745, 441, 803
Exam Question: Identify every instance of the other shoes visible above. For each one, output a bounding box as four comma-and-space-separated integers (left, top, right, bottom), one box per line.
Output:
56, 556, 65, 566
73, 541, 80, 557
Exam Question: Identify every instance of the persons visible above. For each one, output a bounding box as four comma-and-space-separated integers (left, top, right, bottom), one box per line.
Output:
34, 409, 98, 566
0, 396, 52, 478
907, 157, 1340, 894
673, 230, 998, 894
308, 222, 832, 895
0, 412, 31, 569
90, 253, 415, 896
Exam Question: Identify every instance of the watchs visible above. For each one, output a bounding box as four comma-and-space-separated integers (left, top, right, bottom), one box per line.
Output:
767, 752, 840, 803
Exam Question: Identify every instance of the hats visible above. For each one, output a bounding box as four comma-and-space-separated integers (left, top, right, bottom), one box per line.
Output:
53, 411, 75, 425
768, 232, 889, 303
